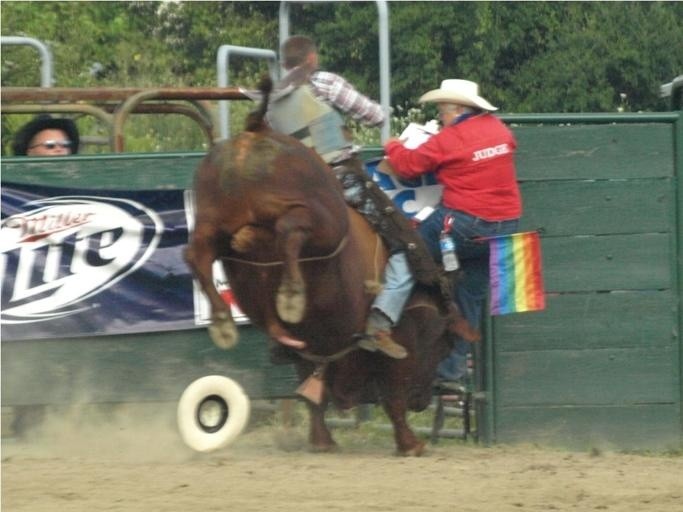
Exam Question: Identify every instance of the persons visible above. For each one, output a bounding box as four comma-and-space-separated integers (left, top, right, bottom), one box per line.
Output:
10, 114, 81, 159
381, 77, 523, 383
264, 34, 418, 361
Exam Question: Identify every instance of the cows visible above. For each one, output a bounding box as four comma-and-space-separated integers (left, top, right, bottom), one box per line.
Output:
179, 70, 482, 460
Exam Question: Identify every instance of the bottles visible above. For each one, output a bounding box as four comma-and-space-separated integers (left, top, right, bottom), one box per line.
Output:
437, 231, 460, 272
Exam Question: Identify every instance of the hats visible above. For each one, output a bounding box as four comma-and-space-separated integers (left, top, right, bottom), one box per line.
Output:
419, 78, 499, 112
14, 115, 80, 156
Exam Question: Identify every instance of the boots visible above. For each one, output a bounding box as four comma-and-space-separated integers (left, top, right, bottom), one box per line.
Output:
359, 307, 407, 359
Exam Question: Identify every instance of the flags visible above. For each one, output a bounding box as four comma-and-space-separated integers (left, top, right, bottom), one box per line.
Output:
485, 229, 544, 316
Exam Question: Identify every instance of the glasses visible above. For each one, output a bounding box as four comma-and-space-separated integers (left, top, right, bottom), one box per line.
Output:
27, 139, 73, 150
